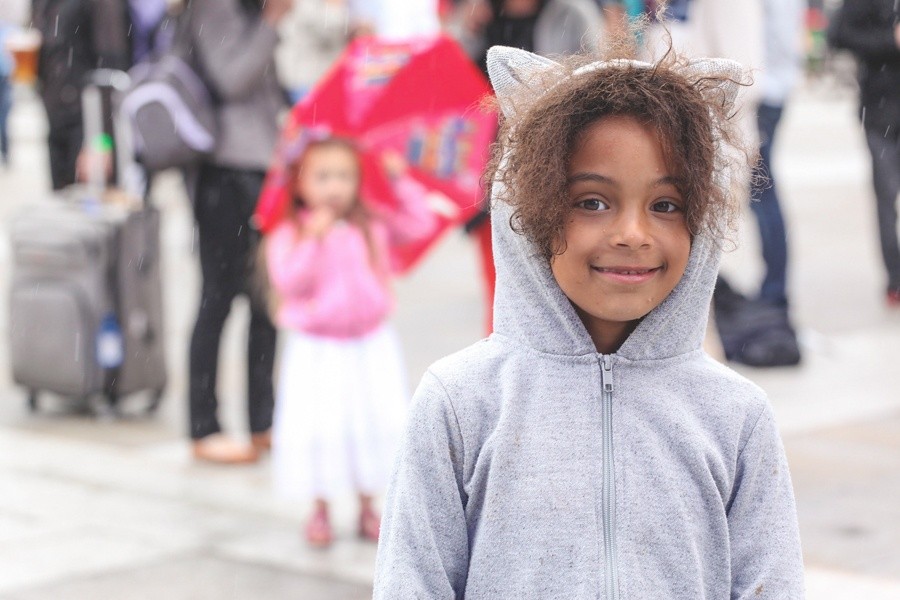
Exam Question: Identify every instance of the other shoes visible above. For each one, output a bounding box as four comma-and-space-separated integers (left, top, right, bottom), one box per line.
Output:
359, 512, 380, 538
195, 432, 240, 462
307, 516, 328, 545
730, 326, 799, 367
240, 427, 271, 462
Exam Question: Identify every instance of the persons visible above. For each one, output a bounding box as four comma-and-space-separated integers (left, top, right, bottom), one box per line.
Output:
0, 0, 900, 464
369, 45, 804, 600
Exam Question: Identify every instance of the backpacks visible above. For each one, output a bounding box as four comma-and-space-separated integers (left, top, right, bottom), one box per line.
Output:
122, 0, 220, 171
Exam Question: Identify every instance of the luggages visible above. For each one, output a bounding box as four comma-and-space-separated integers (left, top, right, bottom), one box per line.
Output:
4, 67, 167, 417
